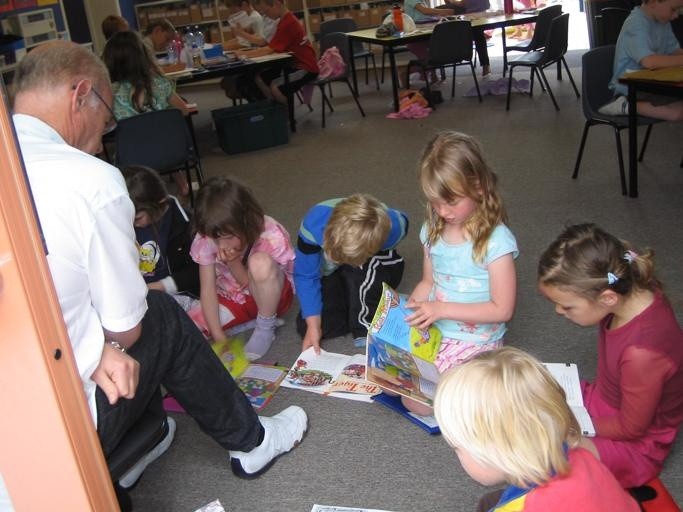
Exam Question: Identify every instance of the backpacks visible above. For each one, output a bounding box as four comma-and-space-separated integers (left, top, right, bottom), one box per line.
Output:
317, 47, 346, 79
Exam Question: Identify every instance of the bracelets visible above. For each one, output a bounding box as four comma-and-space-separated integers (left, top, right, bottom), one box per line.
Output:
104, 338, 126, 353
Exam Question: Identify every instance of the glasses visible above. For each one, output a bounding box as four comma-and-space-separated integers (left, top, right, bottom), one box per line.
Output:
72, 83, 119, 136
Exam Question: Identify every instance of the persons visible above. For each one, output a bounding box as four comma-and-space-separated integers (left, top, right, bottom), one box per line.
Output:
101, 28, 198, 197
100, 13, 129, 42
115, 164, 199, 314
596, 0, 682, 123
399, 128, 521, 378
443, 1, 491, 79
402, 1, 455, 85
536, 219, 682, 489
431, 343, 641, 512
12, 38, 310, 491
219, 0, 265, 103
189, 173, 296, 363
292, 190, 410, 356
138, 16, 187, 75
508, 0, 536, 42
232, 0, 319, 127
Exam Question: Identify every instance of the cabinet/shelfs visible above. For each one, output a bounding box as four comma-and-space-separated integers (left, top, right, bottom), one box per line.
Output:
133, 0, 404, 89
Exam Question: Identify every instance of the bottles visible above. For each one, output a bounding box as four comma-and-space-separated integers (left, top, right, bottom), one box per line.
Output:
391, 3, 403, 34
167, 24, 205, 70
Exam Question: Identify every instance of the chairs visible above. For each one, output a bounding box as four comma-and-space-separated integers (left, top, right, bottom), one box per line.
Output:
378, 11, 447, 83
112, 107, 204, 210
317, 17, 381, 99
570, 43, 666, 198
501, 3, 582, 115
404, 19, 483, 111
299, 30, 365, 127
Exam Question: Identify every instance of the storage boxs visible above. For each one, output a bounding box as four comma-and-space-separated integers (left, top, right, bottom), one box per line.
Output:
207, 99, 288, 155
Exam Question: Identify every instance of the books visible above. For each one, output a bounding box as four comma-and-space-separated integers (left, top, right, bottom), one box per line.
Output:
535, 359, 597, 439
363, 279, 442, 410
207, 333, 291, 418
278, 344, 384, 404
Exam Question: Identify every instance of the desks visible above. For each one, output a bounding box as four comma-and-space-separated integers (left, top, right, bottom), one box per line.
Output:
342, 11, 540, 114
155, 48, 298, 141
616, 63, 682, 198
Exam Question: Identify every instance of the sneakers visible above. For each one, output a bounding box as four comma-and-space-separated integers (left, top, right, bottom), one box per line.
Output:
228, 405, 310, 479
118, 416, 176, 488
599, 95, 627, 115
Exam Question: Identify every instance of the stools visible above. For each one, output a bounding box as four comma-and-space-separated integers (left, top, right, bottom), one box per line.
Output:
103, 406, 167, 511
